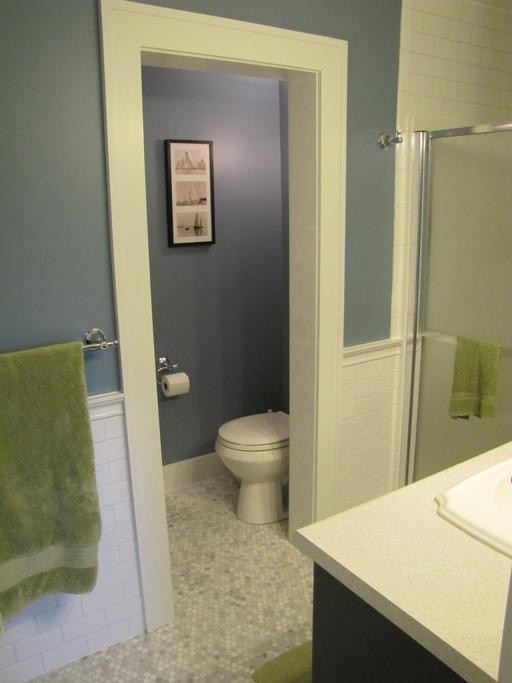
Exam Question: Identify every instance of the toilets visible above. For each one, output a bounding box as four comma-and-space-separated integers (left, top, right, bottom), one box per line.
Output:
214, 409, 289, 524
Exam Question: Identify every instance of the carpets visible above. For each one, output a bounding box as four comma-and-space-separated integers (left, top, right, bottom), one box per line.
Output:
251, 639, 313, 682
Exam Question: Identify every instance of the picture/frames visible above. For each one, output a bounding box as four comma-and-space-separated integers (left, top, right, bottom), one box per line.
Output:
164, 140, 216, 248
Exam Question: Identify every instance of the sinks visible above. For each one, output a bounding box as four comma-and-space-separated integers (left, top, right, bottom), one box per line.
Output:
435, 454, 512, 560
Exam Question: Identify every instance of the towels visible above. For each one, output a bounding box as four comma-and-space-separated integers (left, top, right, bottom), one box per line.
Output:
2, 341, 100, 630
450, 337, 503, 419
